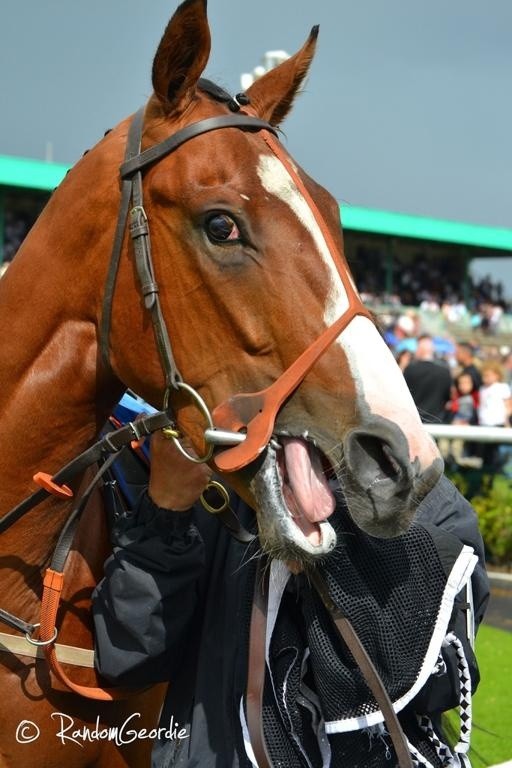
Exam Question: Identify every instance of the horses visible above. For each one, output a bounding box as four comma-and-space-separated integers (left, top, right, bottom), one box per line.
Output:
1, 1, 451, 767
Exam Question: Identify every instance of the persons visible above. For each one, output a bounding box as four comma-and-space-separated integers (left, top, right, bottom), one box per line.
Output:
87, 424, 490, 768
1, 186, 44, 268
344, 229, 512, 466
